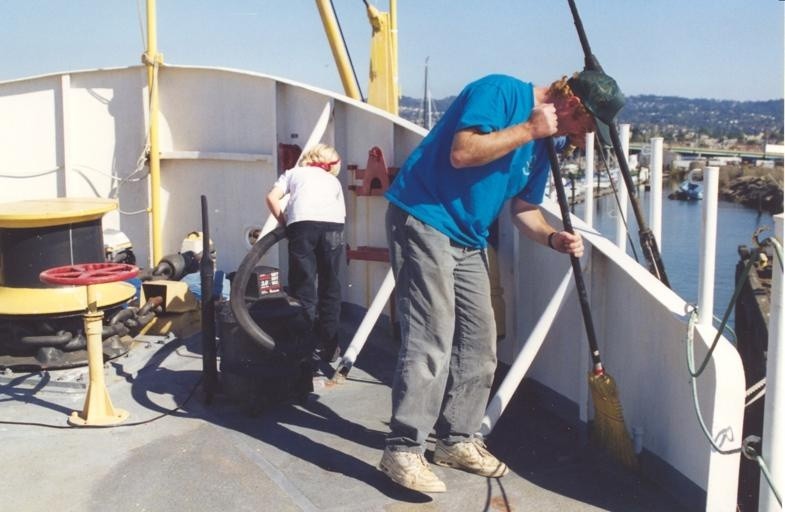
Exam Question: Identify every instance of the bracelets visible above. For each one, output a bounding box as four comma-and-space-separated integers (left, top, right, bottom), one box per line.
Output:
547, 231, 556, 250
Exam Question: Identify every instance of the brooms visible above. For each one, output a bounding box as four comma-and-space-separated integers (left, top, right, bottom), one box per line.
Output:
542, 133, 639, 474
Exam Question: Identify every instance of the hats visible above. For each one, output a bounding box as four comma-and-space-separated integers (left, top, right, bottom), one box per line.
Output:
567, 70, 627, 147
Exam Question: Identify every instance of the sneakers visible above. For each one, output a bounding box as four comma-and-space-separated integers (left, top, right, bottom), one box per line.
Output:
379, 445, 447, 493
434, 436, 509, 477
325, 344, 340, 361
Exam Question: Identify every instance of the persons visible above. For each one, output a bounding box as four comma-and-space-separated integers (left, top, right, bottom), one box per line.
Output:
376, 66, 630, 494
266, 144, 349, 373
